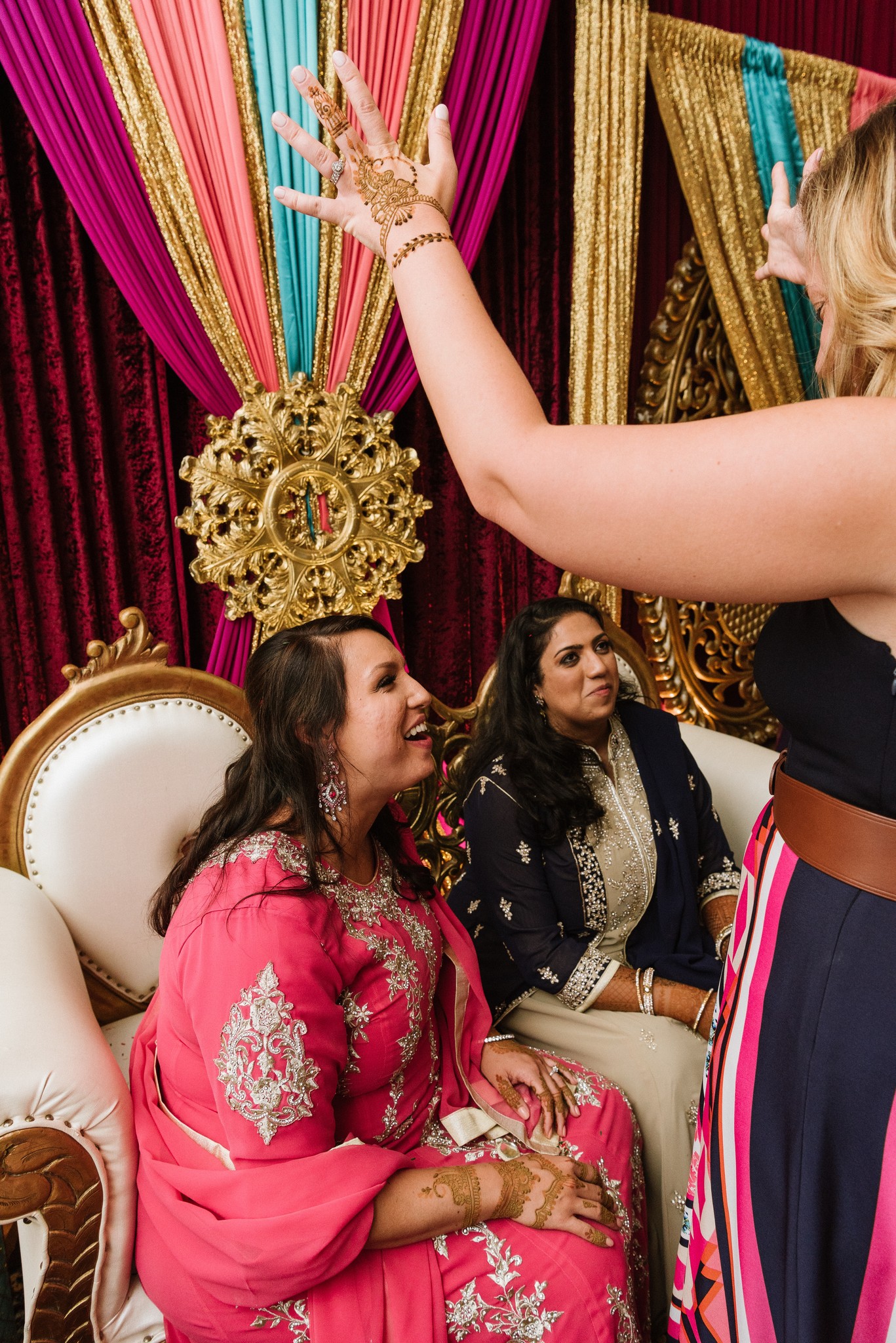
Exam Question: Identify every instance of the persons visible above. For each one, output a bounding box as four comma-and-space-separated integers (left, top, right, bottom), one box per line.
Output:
448, 600, 742, 1341
259, 45, 895, 1343
120, 614, 662, 1343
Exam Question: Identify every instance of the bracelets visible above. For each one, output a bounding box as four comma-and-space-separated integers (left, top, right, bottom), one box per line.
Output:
692, 987, 714, 1032
714, 923, 736, 964
642, 967, 656, 1016
468, 1033, 516, 1046
699, 887, 739, 920
635, 967, 646, 1016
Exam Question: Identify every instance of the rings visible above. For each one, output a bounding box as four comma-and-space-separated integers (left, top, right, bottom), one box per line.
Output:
329, 158, 345, 187
548, 1065, 560, 1077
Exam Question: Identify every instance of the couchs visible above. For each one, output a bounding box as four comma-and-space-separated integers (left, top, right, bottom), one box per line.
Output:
0, 605, 782, 1343
